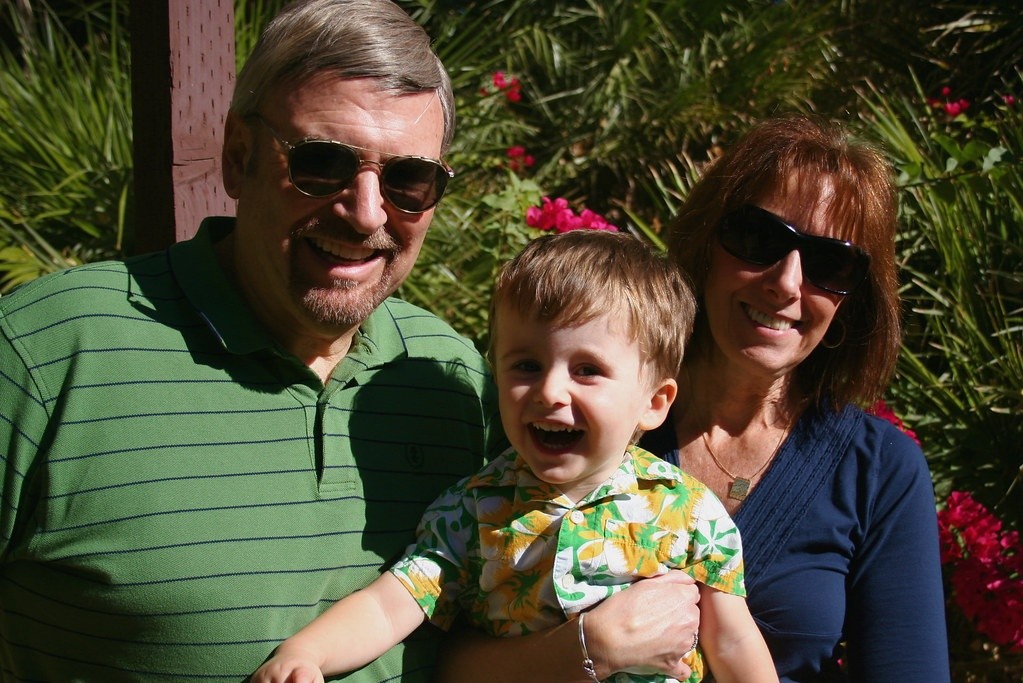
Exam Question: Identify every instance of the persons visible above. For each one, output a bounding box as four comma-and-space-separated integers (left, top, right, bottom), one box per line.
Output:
243, 226, 786, 682
631, 122, 952, 683
1, 0, 504, 683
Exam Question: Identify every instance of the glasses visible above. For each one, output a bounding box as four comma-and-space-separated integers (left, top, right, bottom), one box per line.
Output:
717, 201, 872, 296
246, 110, 455, 214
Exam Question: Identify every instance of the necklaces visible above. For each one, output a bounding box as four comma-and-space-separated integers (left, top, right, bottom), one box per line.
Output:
684, 361, 803, 502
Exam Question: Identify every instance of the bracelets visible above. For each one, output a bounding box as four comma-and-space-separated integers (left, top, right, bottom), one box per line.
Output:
578, 613, 602, 683
